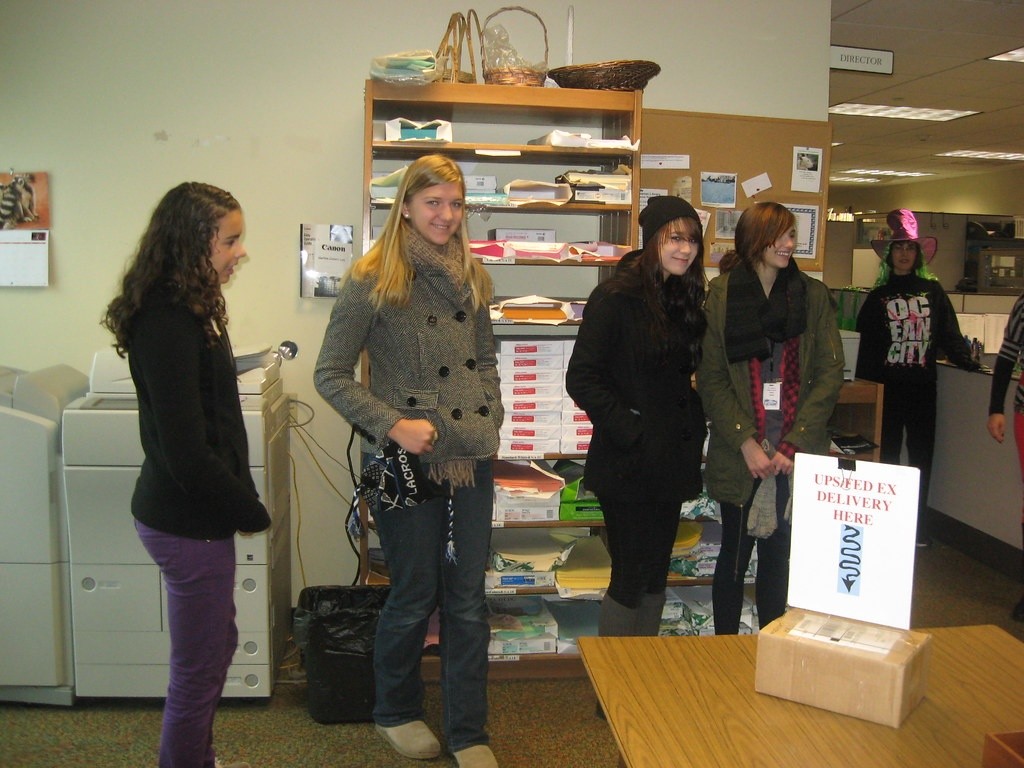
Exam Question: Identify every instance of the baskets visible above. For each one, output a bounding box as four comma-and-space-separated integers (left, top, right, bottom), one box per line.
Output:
478, 4, 551, 88
429, 8, 484, 84
546, 58, 662, 94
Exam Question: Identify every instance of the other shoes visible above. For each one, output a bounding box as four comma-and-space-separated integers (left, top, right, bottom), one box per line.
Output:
1012, 595, 1024, 623
213, 756, 251, 768
916, 534, 929, 548
374, 720, 442, 759
452, 745, 500, 768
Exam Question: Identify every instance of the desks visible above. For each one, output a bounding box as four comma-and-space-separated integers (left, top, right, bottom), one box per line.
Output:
577, 624, 1024, 768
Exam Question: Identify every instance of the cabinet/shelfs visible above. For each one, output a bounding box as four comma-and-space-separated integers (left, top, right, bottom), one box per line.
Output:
361, 81, 643, 679
829, 379, 884, 463
663, 452, 761, 585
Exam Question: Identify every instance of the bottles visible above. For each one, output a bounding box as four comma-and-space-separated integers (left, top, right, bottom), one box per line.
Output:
971, 337, 980, 362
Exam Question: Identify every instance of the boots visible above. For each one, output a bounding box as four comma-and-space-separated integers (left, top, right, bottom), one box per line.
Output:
592, 592, 641, 722
636, 590, 668, 636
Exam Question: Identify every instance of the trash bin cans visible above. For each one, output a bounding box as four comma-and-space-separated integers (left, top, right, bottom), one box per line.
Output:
300, 585, 389, 720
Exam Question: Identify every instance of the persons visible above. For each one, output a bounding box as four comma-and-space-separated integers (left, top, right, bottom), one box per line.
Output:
565, 195, 707, 720
856, 208, 983, 547
312, 156, 505, 768
987, 290, 1024, 619
102, 183, 272, 768
694, 203, 845, 634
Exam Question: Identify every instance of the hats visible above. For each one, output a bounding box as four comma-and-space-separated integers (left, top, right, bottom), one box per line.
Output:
870, 209, 938, 265
637, 195, 702, 250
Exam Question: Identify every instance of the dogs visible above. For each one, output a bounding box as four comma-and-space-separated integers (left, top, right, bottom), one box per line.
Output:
797, 156, 814, 171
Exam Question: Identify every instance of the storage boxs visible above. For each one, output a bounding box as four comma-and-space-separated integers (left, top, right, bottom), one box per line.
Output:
495, 340, 593, 454
488, 229, 556, 242
757, 609, 931, 729
493, 491, 603, 521
494, 571, 556, 587
462, 173, 497, 195
488, 616, 556, 654
